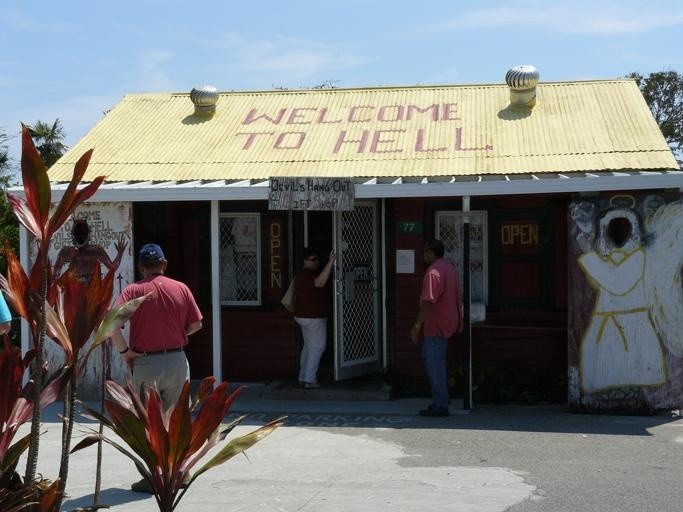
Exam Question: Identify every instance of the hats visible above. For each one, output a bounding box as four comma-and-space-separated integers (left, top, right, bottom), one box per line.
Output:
139, 243, 165, 265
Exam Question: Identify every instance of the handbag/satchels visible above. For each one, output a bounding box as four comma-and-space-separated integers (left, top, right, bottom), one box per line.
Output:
281, 276, 296, 312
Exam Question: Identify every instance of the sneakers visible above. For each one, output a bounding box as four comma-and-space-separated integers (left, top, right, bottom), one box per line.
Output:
298, 381, 321, 389
420, 405, 450, 417
132, 470, 189, 495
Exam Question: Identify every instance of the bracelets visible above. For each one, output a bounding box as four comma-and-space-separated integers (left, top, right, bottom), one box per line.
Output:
411, 325, 421, 332
118, 346, 130, 354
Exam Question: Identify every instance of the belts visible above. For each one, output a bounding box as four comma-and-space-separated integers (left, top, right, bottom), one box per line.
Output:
135, 347, 182, 356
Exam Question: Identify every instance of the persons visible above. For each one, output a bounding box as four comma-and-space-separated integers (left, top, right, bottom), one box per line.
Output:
110, 244, 206, 492
0, 288, 13, 336
293, 248, 336, 390
409, 239, 463, 416
